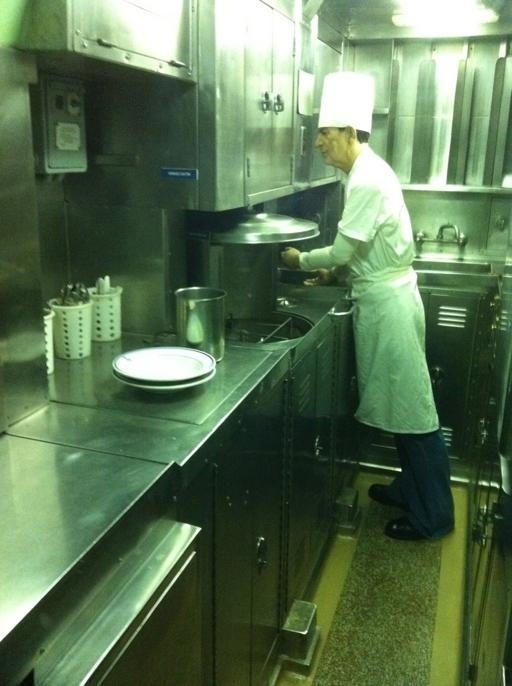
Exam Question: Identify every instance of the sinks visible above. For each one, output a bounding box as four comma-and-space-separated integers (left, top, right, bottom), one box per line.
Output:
414, 252, 491, 270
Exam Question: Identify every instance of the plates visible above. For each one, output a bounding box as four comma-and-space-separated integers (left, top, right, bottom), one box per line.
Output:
111, 347, 217, 395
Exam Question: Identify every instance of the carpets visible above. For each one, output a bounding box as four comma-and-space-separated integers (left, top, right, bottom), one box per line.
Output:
312, 501, 442, 686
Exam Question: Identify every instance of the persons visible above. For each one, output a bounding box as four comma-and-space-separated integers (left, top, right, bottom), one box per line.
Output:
280, 125, 455, 541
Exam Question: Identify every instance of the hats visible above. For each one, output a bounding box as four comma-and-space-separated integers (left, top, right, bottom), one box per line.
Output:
318, 71, 375, 134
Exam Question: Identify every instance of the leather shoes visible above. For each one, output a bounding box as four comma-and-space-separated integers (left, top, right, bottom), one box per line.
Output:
383, 515, 427, 541
368, 483, 404, 506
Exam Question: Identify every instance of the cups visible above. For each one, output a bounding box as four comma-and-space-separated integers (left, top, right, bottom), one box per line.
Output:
173, 285, 227, 363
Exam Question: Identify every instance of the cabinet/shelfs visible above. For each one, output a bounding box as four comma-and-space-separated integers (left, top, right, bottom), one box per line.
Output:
371, 286, 487, 460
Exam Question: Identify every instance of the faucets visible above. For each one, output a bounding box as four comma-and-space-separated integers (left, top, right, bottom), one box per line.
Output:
437, 223, 459, 240
